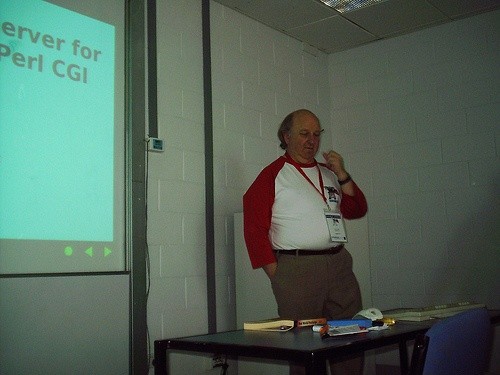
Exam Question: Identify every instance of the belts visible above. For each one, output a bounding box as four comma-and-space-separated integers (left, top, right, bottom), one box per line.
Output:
273, 244, 345, 256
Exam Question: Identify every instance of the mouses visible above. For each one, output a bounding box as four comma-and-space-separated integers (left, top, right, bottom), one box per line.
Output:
352, 307, 383, 322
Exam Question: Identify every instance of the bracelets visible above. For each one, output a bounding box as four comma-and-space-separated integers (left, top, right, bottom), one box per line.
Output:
337, 172, 351, 186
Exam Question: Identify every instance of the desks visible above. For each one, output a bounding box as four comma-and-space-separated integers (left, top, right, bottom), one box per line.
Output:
154, 308, 429, 375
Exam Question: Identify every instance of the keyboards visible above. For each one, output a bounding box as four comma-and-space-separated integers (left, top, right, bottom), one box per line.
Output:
382, 301, 486, 321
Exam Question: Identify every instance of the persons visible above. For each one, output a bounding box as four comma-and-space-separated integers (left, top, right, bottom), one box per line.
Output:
243, 109, 368, 375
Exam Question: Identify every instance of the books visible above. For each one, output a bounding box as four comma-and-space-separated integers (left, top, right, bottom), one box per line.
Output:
243, 318, 297, 333
